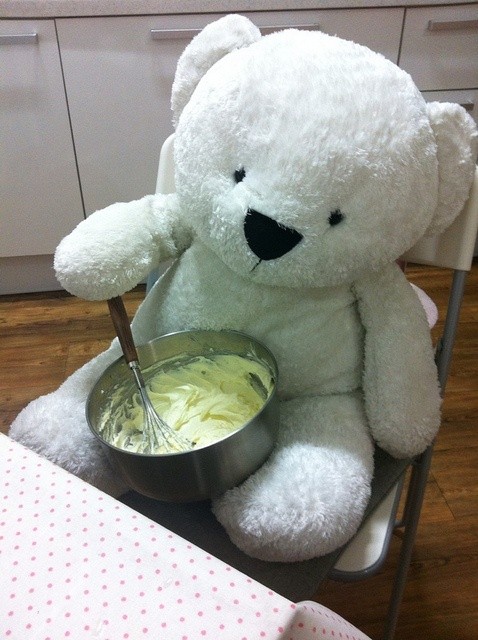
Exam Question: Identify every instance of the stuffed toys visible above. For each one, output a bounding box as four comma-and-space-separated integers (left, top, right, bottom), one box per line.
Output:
10, 12, 477, 563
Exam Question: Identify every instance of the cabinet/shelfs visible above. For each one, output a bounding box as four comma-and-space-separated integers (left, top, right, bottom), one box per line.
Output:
51, 2, 406, 293
399, 3, 478, 112
1, 2, 87, 298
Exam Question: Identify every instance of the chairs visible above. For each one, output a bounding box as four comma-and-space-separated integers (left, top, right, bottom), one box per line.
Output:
110, 131, 477, 640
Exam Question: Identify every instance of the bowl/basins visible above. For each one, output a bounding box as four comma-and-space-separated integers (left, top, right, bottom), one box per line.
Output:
86, 327, 280, 505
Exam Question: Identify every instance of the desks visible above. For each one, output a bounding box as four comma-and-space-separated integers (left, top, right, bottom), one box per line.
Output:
0, 431, 368, 640
